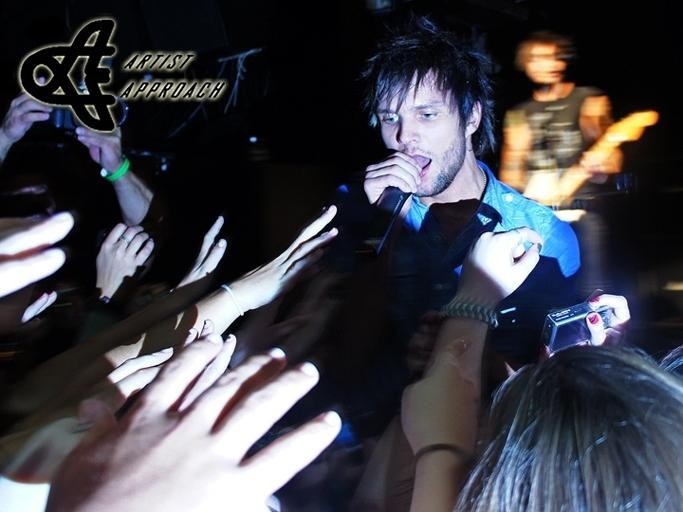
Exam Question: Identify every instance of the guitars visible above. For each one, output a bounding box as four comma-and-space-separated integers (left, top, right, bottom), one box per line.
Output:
520, 111, 659, 207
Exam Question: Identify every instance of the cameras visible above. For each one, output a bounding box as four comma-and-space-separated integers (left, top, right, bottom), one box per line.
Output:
53, 107, 81, 130
540, 301, 613, 353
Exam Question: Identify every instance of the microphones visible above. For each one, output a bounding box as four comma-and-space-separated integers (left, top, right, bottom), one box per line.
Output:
356, 185, 412, 255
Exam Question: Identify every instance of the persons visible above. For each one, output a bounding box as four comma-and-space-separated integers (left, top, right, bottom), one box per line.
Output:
0, 75, 341, 512
500, 31, 624, 297
327, 14, 631, 366
341, 226, 683, 511
